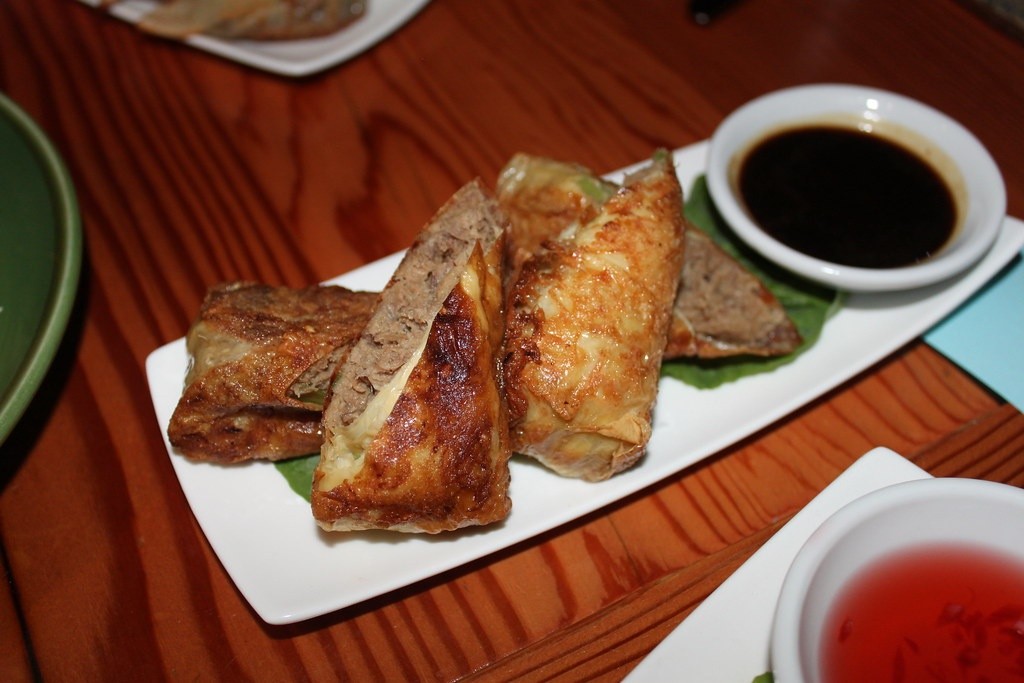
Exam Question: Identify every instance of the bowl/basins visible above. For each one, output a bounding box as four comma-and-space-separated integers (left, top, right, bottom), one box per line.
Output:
705, 82, 1008, 292
765, 477, 1024, 683
0, 92, 86, 450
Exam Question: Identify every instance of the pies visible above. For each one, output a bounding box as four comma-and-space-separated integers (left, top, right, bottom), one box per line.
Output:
160, 148, 803, 533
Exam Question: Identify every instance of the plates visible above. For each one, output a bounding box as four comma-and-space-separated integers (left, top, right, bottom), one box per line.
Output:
146, 135, 1024, 628
614, 446, 937, 683
83, 0, 429, 80
